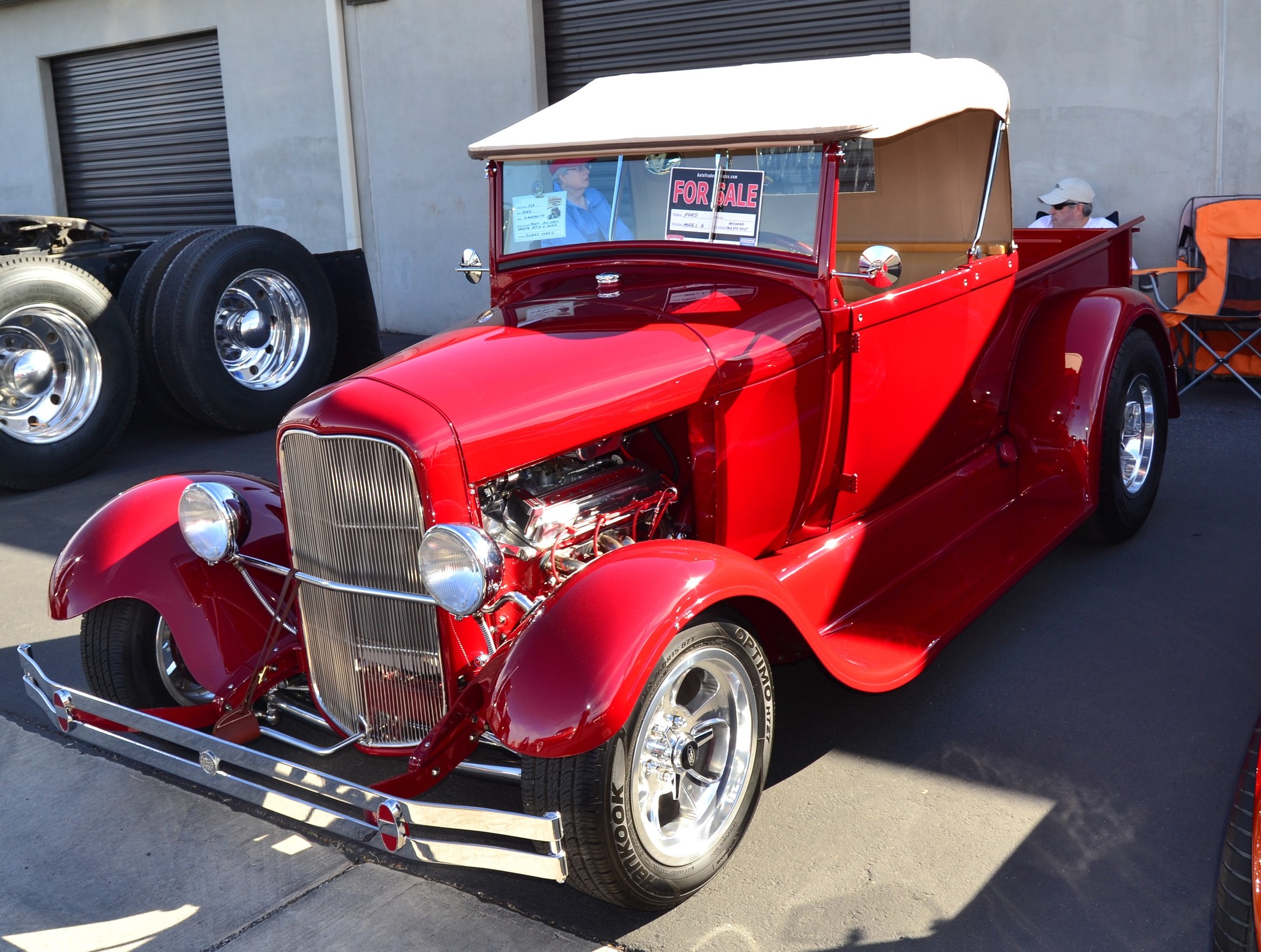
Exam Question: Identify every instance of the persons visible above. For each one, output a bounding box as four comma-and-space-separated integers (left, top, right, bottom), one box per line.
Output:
1028, 178, 1139, 270
540, 157, 635, 249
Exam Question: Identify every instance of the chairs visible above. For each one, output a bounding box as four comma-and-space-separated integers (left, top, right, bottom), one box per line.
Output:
1132, 195, 1261, 398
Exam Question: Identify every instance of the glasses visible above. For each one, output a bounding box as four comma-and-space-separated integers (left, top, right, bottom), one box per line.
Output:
565, 164, 591, 173
1053, 202, 1088, 210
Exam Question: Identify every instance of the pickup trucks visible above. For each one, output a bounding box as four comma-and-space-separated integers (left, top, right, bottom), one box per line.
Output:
16, 55, 1181, 910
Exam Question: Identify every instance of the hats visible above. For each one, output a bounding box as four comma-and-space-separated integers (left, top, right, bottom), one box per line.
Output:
549, 158, 597, 175
1038, 178, 1095, 204
